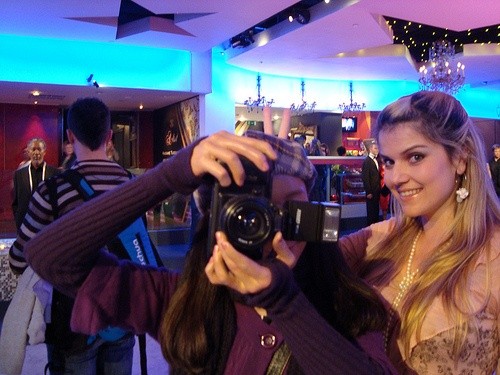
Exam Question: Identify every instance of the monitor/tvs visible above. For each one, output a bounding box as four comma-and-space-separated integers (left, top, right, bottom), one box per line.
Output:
341, 117, 358, 132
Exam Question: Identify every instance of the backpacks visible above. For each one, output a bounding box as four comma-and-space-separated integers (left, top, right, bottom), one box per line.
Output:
62, 169, 165, 344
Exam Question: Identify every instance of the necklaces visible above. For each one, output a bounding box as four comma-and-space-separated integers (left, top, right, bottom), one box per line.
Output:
391, 227, 454, 311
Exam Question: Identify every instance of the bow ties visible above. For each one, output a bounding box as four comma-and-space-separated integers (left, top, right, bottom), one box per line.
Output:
370, 155, 377, 159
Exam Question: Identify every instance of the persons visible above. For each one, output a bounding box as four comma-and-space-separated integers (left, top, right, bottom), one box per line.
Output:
362, 143, 380, 226
22, 128, 419, 375
293, 133, 347, 199
264, 91, 500, 375
488, 144, 500, 200
59, 141, 77, 171
9, 97, 148, 375
11, 138, 59, 236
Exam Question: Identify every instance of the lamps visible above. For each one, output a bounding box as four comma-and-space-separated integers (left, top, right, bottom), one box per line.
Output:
418, 41, 466, 95
290, 80, 317, 115
338, 82, 366, 117
243, 71, 275, 114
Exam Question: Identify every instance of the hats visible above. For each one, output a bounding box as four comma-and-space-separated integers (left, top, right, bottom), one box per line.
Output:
193, 131, 318, 216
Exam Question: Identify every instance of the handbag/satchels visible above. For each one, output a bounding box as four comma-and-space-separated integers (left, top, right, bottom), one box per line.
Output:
32, 175, 57, 323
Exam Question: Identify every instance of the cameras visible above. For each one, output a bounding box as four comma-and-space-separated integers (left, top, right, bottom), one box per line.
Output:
205, 159, 342, 264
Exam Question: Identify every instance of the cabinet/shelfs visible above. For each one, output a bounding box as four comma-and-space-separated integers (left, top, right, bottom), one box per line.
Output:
304, 156, 386, 218
